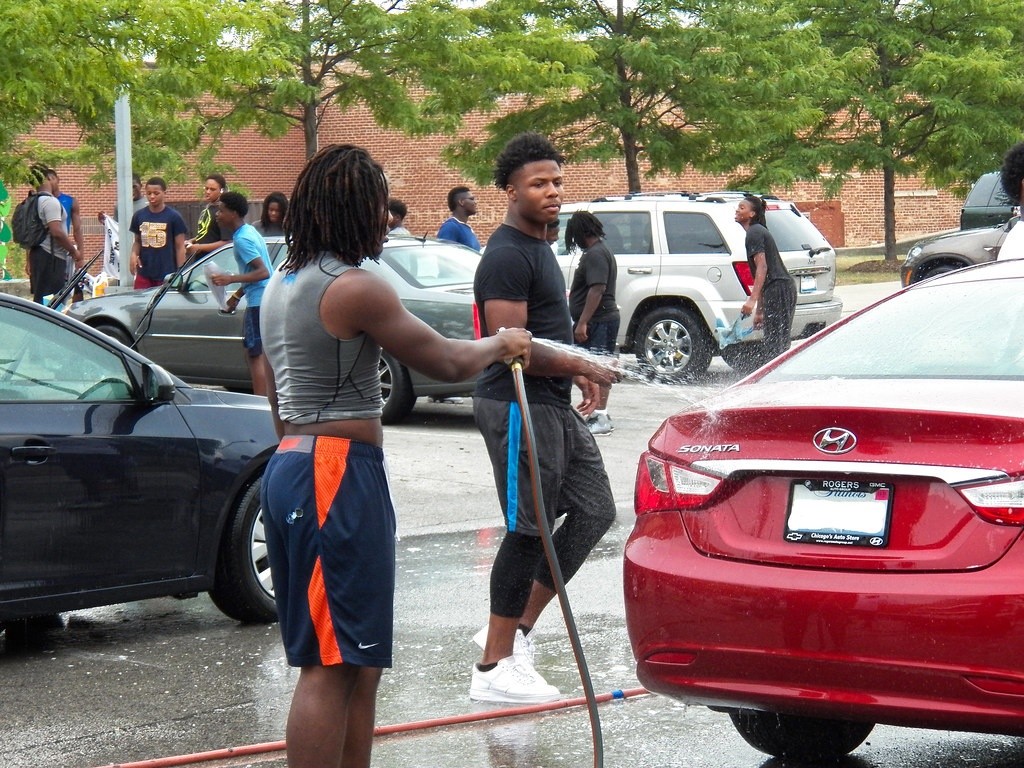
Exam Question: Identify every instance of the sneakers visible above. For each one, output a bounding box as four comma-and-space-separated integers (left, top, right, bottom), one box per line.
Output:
470, 655, 549, 704
473, 623, 535, 668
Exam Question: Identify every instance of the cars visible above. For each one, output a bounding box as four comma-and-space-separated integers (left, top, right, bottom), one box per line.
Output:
0, 292, 281, 625
622, 215, 1024, 761
59, 232, 484, 426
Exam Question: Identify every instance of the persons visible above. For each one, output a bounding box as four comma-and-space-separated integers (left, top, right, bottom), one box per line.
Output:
210, 191, 274, 398
25, 163, 85, 306
564, 211, 622, 437
258, 142, 533, 768
98, 174, 149, 226
436, 187, 480, 255
734, 197, 798, 361
129, 176, 188, 290
546, 217, 560, 246
183, 174, 233, 263
252, 192, 289, 238
996, 142, 1024, 261
469, 133, 617, 709
386, 197, 412, 238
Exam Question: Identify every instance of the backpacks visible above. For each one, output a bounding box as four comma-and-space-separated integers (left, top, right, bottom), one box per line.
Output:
12, 190, 64, 248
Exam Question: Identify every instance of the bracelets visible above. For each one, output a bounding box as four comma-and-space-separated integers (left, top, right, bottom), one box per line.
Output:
232, 293, 241, 302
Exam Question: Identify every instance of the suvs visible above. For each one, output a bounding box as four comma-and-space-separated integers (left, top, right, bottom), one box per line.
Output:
478, 170, 1024, 384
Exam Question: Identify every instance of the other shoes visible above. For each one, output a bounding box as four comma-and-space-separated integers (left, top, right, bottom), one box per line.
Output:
583, 412, 615, 436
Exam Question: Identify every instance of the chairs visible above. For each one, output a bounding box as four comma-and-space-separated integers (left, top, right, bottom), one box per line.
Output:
639, 222, 653, 253
604, 223, 626, 254
